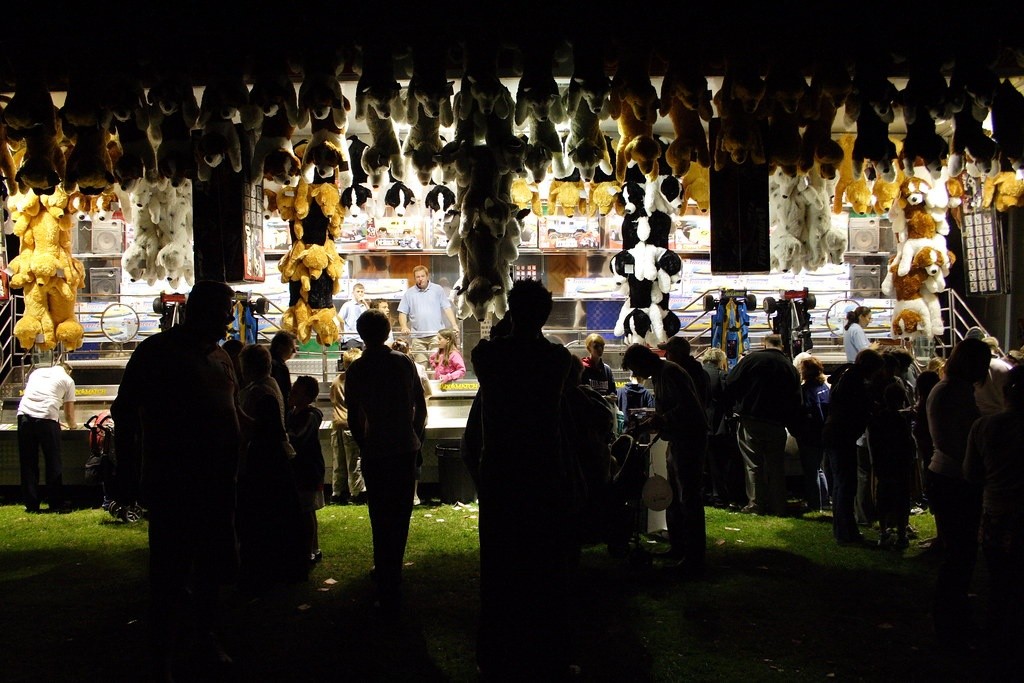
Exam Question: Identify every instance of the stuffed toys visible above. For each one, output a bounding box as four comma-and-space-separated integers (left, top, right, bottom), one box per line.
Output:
612, 164, 680, 349
832, 1, 1024, 343
446, 168, 530, 321
120, 176, 195, 290
0, 0, 843, 220
5, 184, 84, 353
274, 137, 344, 347
775, 161, 847, 270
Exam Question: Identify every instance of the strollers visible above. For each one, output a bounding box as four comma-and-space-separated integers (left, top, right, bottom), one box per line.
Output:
84, 415, 143, 523
608, 413, 662, 560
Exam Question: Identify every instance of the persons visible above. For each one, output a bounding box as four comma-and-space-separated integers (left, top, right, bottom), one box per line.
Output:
346, 307, 427, 592
461, 278, 622, 683
397, 263, 460, 370
110, 279, 325, 683
428, 327, 465, 386
621, 346, 710, 571
16, 362, 77, 514
844, 306, 883, 363
329, 347, 367, 506
616, 374, 656, 471
336, 283, 370, 362
391, 339, 431, 506
579, 337, 618, 409
371, 299, 394, 349
659, 334, 1023, 659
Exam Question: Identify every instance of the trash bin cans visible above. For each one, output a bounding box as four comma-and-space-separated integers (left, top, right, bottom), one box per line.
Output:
296, 331, 340, 359
434, 442, 476, 501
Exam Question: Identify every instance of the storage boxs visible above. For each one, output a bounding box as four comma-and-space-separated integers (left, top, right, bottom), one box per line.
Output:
668, 259, 895, 330
562, 277, 620, 298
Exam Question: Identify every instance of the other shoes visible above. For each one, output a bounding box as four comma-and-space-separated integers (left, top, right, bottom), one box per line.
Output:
843, 524, 918, 551
